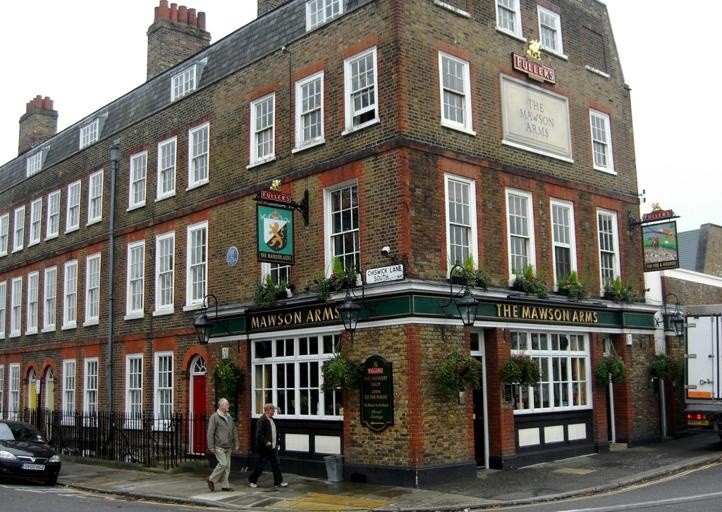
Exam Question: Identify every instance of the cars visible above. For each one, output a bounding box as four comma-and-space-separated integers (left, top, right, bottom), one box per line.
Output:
0, 421, 61, 486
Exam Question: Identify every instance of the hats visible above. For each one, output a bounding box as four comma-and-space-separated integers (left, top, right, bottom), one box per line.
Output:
264, 404, 277, 411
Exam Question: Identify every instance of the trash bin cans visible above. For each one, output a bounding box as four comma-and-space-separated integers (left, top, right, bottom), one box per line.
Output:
322, 455, 344, 482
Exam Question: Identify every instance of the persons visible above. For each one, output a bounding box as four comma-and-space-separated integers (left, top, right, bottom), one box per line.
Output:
245, 403, 290, 488
205, 396, 238, 492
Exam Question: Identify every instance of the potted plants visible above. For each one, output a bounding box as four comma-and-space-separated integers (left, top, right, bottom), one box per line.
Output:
597, 353, 622, 381
320, 354, 361, 394
309, 255, 358, 299
649, 353, 686, 383
604, 276, 638, 305
425, 351, 484, 394
557, 271, 587, 301
214, 355, 243, 399
252, 275, 294, 305
451, 255, 484, 285
499, 354, 541, 391
511, 264, 549, 299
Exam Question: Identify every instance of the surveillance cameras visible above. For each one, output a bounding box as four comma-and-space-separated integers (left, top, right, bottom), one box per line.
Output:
381, 246, 390, 257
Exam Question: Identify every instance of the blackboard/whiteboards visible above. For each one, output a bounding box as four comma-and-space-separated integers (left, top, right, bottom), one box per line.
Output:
359, 355, 394, 433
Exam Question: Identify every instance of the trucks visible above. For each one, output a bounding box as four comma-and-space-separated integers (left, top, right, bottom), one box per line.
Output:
683, 312, 722, 441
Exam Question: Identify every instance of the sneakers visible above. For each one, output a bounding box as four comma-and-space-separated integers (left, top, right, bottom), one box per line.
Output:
275, 482, 288, 488
221, 487, 235, 492
207, 478, 214, 492
248, 482, 258, 488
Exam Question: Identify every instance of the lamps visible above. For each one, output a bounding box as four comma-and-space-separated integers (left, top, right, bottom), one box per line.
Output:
662, 292, 686, 337
193, 293, 233, 345
336, 284, 361, 345
450, 265, 479, 329
